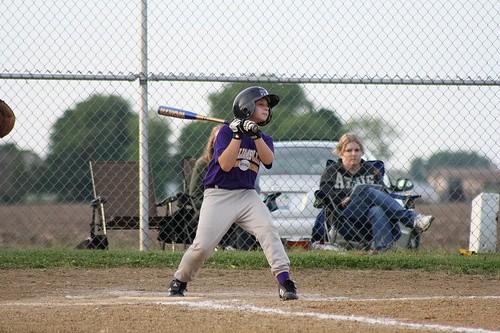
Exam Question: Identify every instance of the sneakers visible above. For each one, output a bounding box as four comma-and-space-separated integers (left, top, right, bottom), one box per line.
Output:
278, 279, 299, 301
413, 214, 434, 232
167, 279, 188, 297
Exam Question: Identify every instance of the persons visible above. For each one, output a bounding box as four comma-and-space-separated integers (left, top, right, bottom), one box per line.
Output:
320, 132, 434, 255
168, 86, 298, 300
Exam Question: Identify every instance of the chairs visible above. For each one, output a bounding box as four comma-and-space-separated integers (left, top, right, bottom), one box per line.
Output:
182, 155, 281, 250
89, 160, 177, 253
314, 159, 421, 254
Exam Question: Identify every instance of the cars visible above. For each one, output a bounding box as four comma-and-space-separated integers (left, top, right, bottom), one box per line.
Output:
253, 140, 420, 253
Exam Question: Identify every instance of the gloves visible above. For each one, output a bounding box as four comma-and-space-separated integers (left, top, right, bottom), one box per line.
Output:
238, 119, 261, 140
228, 117, 243, 140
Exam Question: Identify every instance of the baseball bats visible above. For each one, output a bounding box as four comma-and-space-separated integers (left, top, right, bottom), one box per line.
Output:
157, 105, 259, 133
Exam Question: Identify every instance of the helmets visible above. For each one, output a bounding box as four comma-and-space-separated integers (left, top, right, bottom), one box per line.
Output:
232, 85, 280, 126
